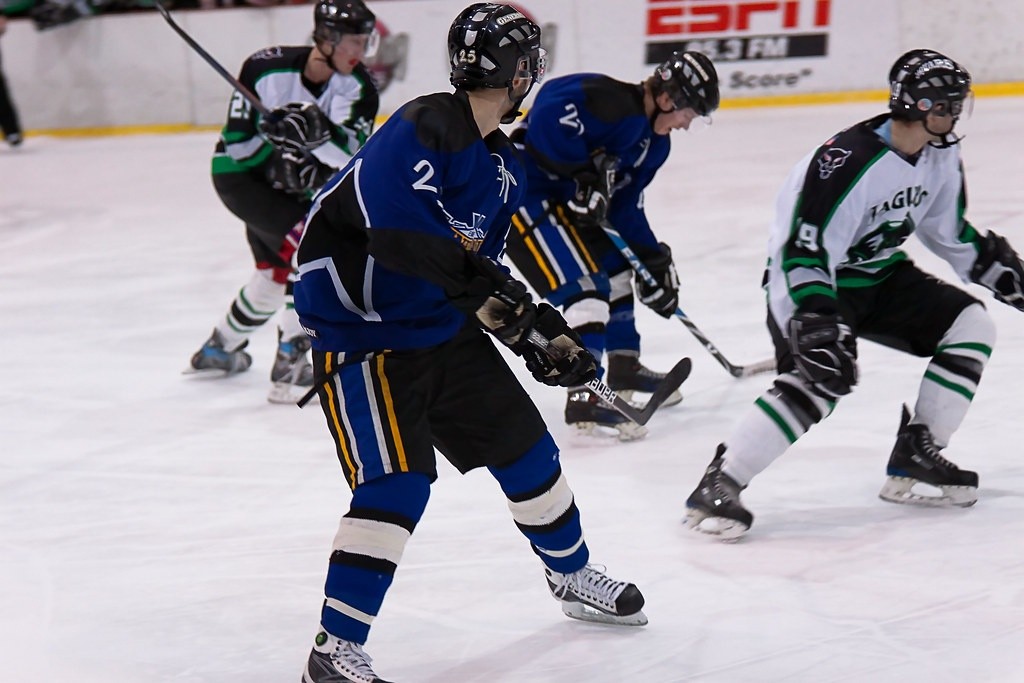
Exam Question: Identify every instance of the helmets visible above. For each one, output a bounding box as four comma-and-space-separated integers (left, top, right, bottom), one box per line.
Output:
447, 3, 547, 102
656, 51, 720, 116
315, 0, 376, 57
888, 49, 974, 122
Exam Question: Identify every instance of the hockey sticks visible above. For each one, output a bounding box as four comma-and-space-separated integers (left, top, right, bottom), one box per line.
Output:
598, 217, 777, 379
526, 328, 693, 425
147, 1, 268, 111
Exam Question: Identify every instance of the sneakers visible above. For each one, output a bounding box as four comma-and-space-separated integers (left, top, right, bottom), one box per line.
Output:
542, 561, 648, 626
267, 324, 320, 403
301, 624, 392, 683
564, 387, 648, 440
181, 327, 251, 374
606, 355, 683, 408
878, 403, 979, 508
680, 443, 753, 544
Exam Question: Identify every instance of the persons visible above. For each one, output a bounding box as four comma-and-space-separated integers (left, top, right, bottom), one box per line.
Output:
0, 14, 22, 145
505, 48, 721, 426
687, 49, 1024, 529
191, 0, 381, 387
286, 2, 646, 682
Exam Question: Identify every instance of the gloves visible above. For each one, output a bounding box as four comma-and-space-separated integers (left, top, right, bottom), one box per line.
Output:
635, 242, 680, 319
275, 151, 339, 195
567, 172, 607, 228
788, 294, 858, 396
257, 101, 330, 150
444, 251, 536, 344
969, 229, 1024, 312
521, 303, 597, 386
